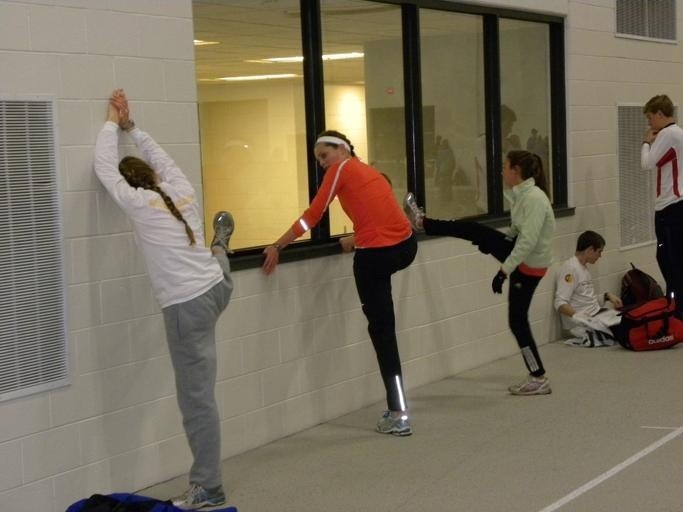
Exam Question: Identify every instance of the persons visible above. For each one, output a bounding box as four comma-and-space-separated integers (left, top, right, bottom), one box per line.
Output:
260, 130, 418, 438
403, 150, 556, 396
528, 129, 550, 171
92, 88, 234, 512
552, 229, 623, 349
474, 105, 515, 210
432, 135, 454, 198
640, 94, 683, 324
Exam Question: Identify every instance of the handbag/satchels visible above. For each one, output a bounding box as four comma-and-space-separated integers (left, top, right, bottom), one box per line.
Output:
621, 298, 683, 353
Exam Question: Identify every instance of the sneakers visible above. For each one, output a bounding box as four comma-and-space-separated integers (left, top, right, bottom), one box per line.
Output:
172, 485, 226, 510
402, 191, 427, 233
512, 377, 555, 396
211, 208, 235, 253
373, 413, 412, 434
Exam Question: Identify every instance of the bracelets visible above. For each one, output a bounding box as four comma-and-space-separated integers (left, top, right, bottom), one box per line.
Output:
272, 241, 283, 250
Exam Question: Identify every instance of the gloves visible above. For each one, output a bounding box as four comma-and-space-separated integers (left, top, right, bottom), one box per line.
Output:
489, 272, 507, 295
